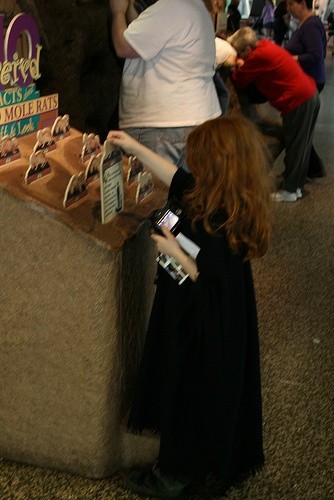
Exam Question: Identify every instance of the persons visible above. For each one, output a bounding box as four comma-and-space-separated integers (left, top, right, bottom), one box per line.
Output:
207, 0, 301, 50
228, 27, 320, 203
275, 0, 327, 185
107, 115, 273, 500
109, 0, 223, 179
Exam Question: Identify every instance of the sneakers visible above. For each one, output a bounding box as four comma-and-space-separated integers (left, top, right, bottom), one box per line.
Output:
270, 190, 297, 202
297, 188, 303, 198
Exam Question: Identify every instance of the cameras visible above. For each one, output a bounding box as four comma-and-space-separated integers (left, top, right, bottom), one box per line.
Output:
151, 208, 181, 234
